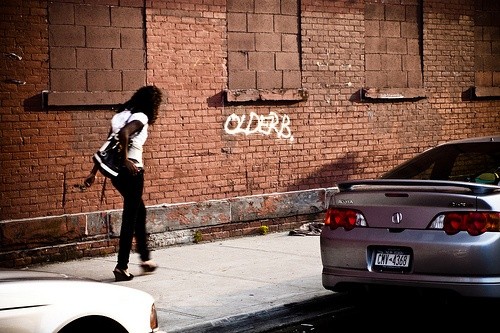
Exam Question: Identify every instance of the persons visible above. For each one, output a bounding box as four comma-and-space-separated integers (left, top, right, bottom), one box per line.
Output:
84, 86, 163, 281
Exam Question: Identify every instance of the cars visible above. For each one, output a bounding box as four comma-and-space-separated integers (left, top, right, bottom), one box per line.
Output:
0, 268, 163, 333
318, 135, 499, 303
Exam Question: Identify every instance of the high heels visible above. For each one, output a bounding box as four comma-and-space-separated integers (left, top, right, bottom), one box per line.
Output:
140, 264, 158, 273
112, 267, 134, 282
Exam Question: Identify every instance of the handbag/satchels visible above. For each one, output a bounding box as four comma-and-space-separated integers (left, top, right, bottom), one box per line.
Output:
91, 112, 142, 179
